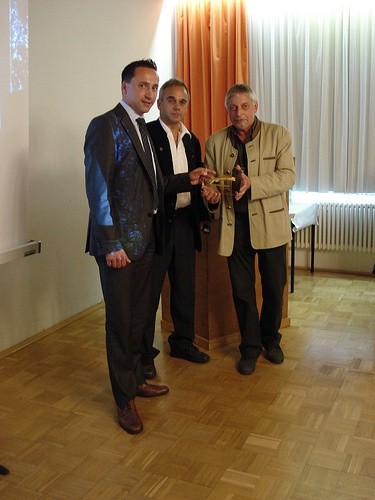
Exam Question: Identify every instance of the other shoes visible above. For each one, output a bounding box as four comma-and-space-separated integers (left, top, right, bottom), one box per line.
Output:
264, 333, 284, 363
239, 357, 258, 375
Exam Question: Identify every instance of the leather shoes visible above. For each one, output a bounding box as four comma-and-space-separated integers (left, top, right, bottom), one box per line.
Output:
144, 364, 156, 379
135, 382, 169, 396
118, 399, 143, 434
170, 341, 211, 363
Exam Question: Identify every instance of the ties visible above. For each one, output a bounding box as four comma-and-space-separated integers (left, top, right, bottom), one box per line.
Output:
136, 118, 159, 211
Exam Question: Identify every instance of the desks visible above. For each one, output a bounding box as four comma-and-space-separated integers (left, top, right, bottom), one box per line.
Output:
289, 201, 318, 293
156, 213, 292, 348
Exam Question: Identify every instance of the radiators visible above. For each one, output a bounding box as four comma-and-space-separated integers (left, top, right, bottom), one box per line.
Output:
292, 198, 375, 253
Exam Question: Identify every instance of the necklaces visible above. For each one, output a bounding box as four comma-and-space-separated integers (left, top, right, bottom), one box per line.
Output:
174, 136, 178, 141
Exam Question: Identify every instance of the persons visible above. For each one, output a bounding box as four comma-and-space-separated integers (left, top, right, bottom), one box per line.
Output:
202, 83, 297, 375
84, 59, 216, 434
145, 78, 211, 379
